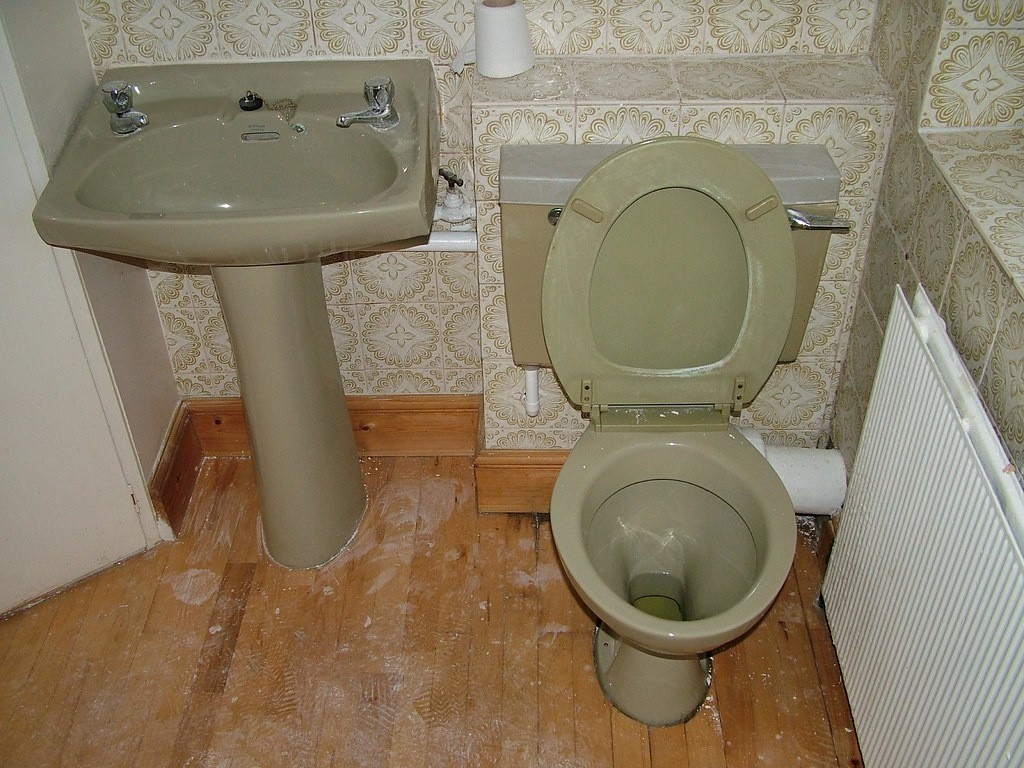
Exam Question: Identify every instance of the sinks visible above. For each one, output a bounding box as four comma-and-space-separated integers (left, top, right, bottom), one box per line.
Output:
31, 59, 440, 267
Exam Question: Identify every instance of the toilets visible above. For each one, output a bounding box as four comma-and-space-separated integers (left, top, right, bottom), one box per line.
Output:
498, 134, 847, 727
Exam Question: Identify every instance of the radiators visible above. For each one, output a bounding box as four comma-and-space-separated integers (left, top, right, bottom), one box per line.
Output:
821, 284, 1024, 768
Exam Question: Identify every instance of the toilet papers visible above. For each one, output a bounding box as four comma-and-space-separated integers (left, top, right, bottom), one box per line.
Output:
450, 1, 535, 77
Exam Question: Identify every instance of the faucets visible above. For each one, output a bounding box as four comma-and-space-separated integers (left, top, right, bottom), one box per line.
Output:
336, 75, 399, 130
102, 80, 150, 135
